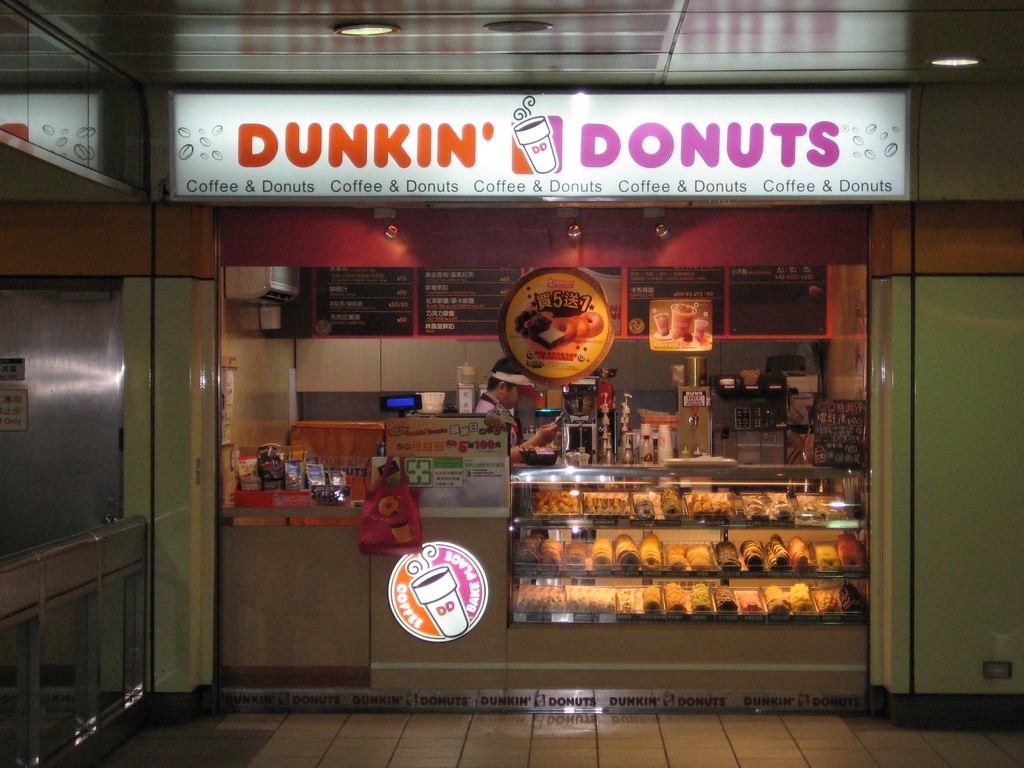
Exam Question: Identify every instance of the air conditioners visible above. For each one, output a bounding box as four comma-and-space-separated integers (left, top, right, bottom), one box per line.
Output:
223, 266, 299, 306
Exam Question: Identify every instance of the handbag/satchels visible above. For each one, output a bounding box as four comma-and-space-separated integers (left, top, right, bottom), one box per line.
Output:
356, 457, 424, 556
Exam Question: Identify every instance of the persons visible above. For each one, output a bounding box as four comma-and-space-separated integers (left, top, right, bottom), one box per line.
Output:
475, 357, 559, 472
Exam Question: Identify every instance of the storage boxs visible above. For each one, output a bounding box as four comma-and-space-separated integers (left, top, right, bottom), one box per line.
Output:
639, 412, 677, 430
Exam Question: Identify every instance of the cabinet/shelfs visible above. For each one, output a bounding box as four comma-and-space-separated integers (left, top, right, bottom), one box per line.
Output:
509, 463, 869, 627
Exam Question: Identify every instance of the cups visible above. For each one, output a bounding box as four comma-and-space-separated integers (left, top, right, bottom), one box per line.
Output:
671, 303, 697, 339
694, 320, 709, 342
641, 424, 654, 456
657, 424, 672, 464
654, 313, 670, 336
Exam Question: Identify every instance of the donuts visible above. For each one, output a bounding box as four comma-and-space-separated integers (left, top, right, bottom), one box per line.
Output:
511, 490, 869, 613
549, 311, 604, 342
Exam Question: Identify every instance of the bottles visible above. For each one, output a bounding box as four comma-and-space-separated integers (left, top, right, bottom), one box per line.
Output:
622, 448, 634, 464
641, 435, 653, 465
694, 446, 701, 458
682, 446, 688, 458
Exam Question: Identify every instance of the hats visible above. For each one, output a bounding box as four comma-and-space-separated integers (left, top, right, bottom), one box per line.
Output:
493, 371, 544, 401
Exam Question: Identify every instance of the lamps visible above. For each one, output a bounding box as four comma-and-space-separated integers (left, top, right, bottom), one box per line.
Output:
385, 209, 401, 240
656, 208, 671, 241
567, 209, 583, 240
